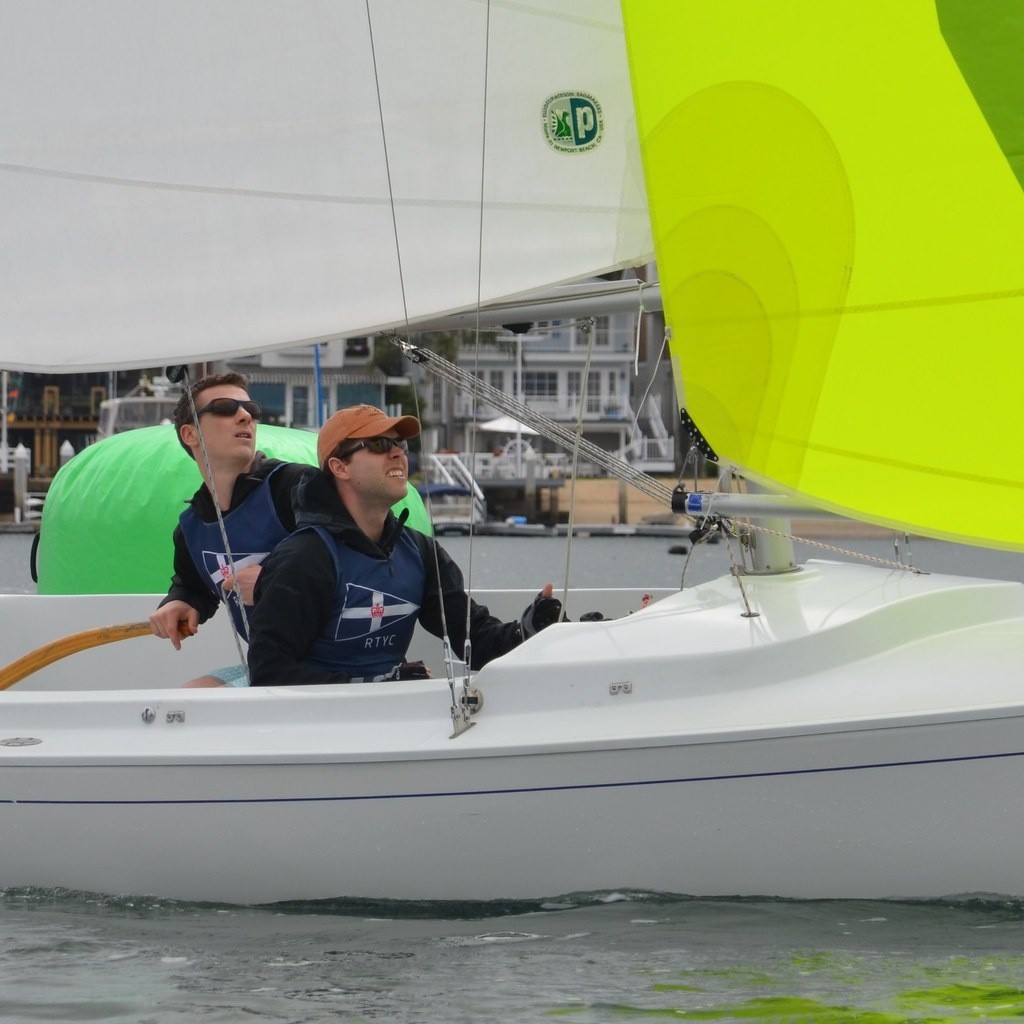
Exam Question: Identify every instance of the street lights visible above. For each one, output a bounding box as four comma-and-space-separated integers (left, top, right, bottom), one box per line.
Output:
496, 336, 545, 480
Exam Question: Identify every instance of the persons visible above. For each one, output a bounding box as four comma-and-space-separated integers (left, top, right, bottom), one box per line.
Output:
247, 404, 571, 686
149, 373, 322, 689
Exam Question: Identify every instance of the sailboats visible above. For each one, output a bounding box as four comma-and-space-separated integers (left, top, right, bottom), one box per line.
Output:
0, 0, 1024, 913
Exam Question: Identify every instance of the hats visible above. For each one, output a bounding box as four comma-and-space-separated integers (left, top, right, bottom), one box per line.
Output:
318, 405, 421, 472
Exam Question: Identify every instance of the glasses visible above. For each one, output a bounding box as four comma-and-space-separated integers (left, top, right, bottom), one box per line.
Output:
185, 397, 261, 424
339, 436, 409, 459
